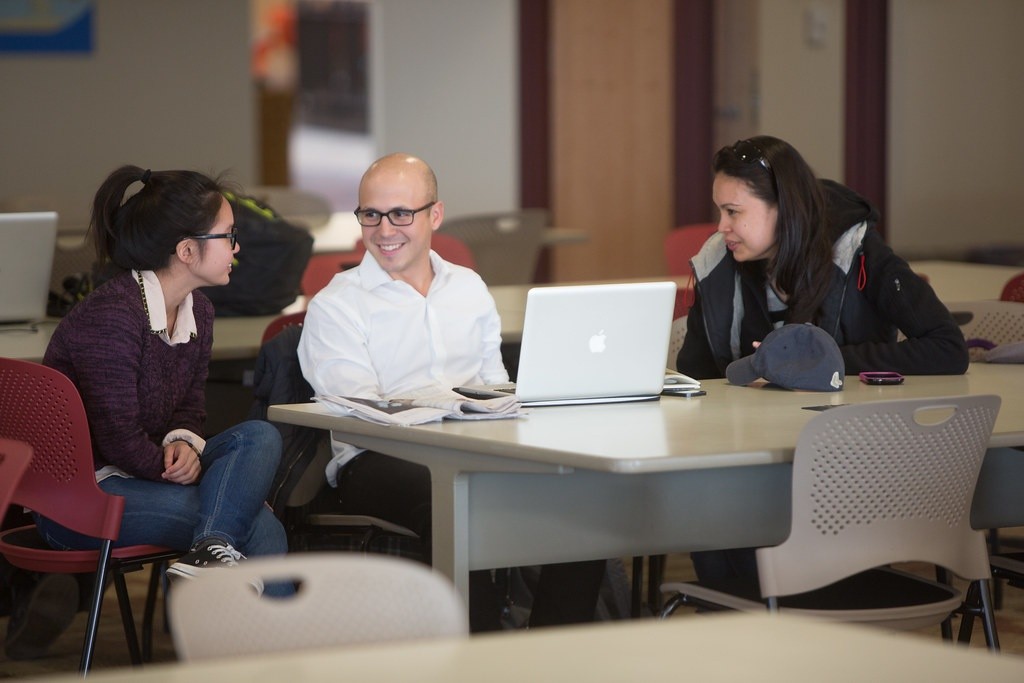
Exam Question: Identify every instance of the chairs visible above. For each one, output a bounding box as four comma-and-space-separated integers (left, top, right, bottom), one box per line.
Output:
1, 271, 1024, 679
439, 209, 544, 285
666, 221, 718, 275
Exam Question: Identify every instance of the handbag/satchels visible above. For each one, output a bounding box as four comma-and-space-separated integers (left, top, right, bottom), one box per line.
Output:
199, 190, 314, 316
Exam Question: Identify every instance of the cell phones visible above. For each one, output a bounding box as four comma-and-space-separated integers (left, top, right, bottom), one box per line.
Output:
663, 391, 706, 397
858, 372, 906, 385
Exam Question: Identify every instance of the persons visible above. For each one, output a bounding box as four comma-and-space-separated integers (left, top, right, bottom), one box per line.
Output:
34, 165, 298, 602
297, 154, 607, 632
678, 139, 969, 616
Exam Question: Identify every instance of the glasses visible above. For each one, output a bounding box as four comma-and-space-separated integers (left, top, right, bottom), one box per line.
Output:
732, 140, 775, 178
168, 228, 239, 250
354, 201, 435, 227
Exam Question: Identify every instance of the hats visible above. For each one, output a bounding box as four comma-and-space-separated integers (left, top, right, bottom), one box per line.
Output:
726, 322, 845, 393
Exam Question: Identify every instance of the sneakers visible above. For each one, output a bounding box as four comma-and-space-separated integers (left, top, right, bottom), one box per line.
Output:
164, 543, 264, 598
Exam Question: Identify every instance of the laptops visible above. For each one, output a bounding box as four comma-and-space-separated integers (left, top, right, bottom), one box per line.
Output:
0, 211, 58, 325
451, 281, 678, 407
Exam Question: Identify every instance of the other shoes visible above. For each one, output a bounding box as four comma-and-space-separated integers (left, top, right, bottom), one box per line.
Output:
5, 572, 79, 660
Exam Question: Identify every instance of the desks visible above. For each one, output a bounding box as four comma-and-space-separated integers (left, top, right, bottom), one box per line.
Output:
35, 609, 1023, 683
0, 259, 1024, 620
